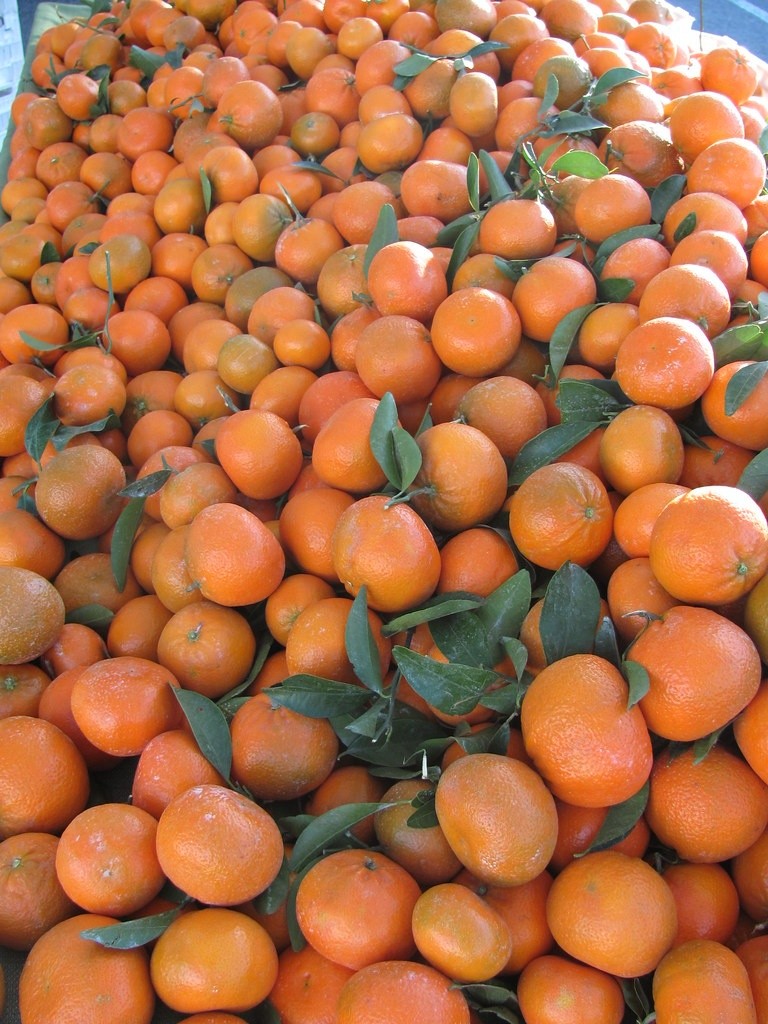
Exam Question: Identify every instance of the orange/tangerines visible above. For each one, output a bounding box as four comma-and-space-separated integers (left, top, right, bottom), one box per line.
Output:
0, 0, 768, 1024
